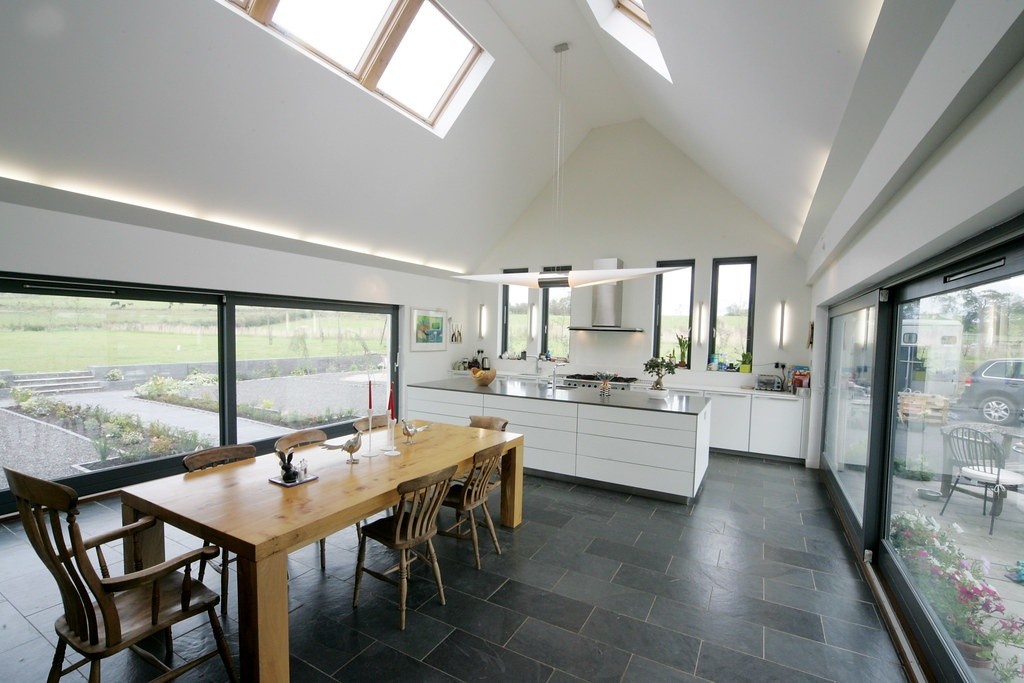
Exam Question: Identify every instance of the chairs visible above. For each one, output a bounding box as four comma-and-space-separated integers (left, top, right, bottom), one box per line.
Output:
939, 426, 1024, 535
1, 415, 510, 682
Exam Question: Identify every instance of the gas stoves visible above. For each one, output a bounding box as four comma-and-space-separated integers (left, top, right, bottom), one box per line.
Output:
566, 374, 638, 385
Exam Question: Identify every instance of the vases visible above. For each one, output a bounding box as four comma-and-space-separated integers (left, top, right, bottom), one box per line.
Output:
599, 386, 612, 397
954, 640, 994, 669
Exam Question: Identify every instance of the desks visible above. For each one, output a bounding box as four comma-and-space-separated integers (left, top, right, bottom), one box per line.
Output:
120, 419, 527, 683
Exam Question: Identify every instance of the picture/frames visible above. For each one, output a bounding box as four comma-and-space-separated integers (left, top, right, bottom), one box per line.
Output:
411, 307, 448, 352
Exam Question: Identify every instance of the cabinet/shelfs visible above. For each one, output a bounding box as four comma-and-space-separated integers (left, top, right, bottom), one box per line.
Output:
629, 384, 811, 464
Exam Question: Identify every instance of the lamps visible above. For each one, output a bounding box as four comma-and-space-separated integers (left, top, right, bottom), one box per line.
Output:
448, 40, 685, 289
778, 300, 786, 349
478, 304, 487, 339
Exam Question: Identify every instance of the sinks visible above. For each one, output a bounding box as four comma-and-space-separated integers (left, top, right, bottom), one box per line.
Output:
519, 373, 541, 376
547, 385, 578, 391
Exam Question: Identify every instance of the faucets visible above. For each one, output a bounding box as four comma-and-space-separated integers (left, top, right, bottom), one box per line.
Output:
552, 363, 566, 390
526, 355, 542, 373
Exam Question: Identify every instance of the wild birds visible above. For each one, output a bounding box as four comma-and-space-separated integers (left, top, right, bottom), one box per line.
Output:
400, 418, 433, 445
319, 431, 364, 464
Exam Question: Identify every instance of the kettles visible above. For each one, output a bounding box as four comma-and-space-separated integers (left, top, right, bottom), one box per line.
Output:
482, 357, 490, 370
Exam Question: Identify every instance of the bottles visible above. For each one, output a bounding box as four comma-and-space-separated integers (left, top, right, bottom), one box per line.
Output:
295, 458, 308, 481
463, 357, 479, 370
792, 370, 803, 395
452, 331, 461, 343
546, 349, 550, 361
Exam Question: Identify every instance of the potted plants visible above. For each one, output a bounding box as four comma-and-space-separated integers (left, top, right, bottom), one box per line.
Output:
642, 333, 689, 399
737, 352, 753, 374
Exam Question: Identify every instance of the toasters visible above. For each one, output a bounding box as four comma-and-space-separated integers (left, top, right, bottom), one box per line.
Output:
757, 374, 783, 391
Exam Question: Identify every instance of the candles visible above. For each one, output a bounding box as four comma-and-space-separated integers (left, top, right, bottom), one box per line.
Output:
388, 381, 395, 419
369, 381, 372, 407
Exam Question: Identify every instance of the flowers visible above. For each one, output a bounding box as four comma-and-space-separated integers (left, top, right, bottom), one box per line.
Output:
889, 510, 1024, 683
594, 371, 617, 387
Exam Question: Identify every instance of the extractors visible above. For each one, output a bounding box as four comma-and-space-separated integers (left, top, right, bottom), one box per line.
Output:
567, 258, 644, 332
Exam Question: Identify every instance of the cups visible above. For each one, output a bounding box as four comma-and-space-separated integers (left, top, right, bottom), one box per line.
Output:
708, 363, 719, 371
797, 387, 811, 398
